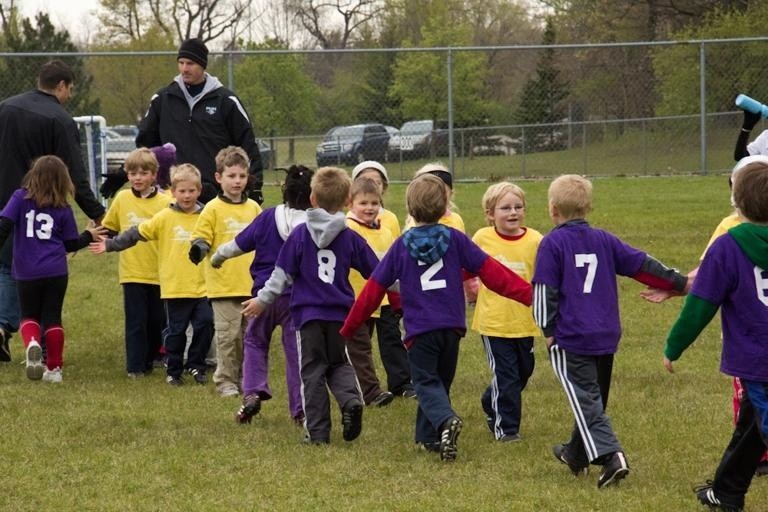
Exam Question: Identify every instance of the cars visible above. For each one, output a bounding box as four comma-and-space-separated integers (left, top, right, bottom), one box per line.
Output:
255, 137, 276, 170
384, 119, 587, 158
75, 123, 140, 181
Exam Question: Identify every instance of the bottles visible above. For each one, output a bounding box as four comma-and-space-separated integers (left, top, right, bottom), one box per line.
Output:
734, 93, 767, 119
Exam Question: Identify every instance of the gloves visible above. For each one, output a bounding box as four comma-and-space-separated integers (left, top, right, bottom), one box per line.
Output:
99, 165, 129, 200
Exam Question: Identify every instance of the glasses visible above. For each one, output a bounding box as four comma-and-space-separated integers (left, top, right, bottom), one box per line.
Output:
493, 203, 525, 213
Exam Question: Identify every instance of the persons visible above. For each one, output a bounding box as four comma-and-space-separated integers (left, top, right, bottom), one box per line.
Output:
640, 154, 768, 511
1, 59, 107, 361
190, 146, 264, 397
471, 182, 545, 443
734, 110, 768, 161
0, 155, 111, 385
339, 174, 534, 463
344, 161, 480, 407
532, 174, 695, 489
211, 165, 314, 430
240, 167, 400, 445
135, 39, 263, 371
101, 148, 176, 379
88, 162, 215, 386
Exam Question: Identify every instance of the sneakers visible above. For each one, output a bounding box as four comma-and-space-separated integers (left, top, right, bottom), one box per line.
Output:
480, 397, 497, 434
692, 478, 746, 511
596, 451, 631, 491
499, 431, 522, 445
125, 351, 416, 447
41, 365, 64, 384
0, 324, 12, 363
416, 438, 441, 453
25, 336, 44, 381
440, 415, 463, 464
552, 442, 590, 480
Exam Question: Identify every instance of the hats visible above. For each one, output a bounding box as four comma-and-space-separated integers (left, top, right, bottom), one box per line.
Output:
174, 37, 211, 70
351, 159, 389, 186
412, 162, 452, 178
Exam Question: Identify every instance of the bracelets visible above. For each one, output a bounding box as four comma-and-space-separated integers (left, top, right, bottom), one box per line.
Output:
741, 128, 751, 132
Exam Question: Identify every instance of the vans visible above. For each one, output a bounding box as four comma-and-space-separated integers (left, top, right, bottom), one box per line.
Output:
315, 124, 390, 168
